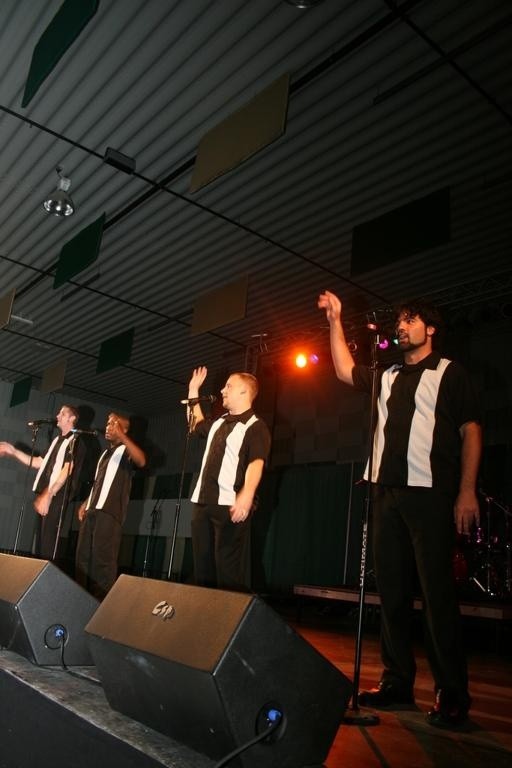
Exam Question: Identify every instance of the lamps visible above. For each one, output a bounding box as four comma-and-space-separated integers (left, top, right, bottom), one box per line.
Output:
244, 300, 403, 370
41, 166, 76, 218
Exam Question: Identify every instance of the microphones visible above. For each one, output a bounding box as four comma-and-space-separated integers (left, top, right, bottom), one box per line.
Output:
70, 428, 100, 437
180, 394, 218, 404
27, 418, 53, 426
368, 322, 399, 339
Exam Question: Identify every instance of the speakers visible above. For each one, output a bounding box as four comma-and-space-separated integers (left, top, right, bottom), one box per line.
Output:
0, 552, 100, 666
83, 572, 354, 767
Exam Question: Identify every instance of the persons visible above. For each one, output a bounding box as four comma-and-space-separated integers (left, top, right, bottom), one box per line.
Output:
1, 402, 87, 569
316, 287, 486, 731
74, 413, 149, 605
186, 365, 273, 592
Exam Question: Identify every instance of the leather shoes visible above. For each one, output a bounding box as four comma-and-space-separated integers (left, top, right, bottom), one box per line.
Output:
357, 675, 415, 710
426, 691, 471, 727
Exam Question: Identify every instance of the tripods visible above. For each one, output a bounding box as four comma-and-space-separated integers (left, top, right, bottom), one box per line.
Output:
467, 500, 512, 605
